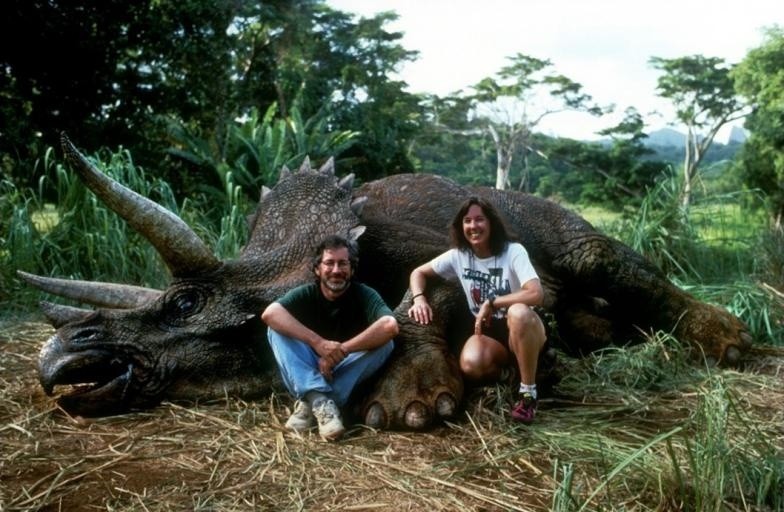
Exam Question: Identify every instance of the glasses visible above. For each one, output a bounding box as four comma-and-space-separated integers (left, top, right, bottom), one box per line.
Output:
321, 260, 350, 266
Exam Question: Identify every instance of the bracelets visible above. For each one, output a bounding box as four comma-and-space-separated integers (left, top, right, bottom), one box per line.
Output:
410, 293, 423, 304
488, 294, 495, 308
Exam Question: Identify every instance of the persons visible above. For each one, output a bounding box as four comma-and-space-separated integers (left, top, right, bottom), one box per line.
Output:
259, 236, 399, 443
408, 197, 551, 424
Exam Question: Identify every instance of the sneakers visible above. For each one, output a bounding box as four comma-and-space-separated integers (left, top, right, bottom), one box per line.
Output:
512, 392, 537, 423
284, 396, 346, 439
